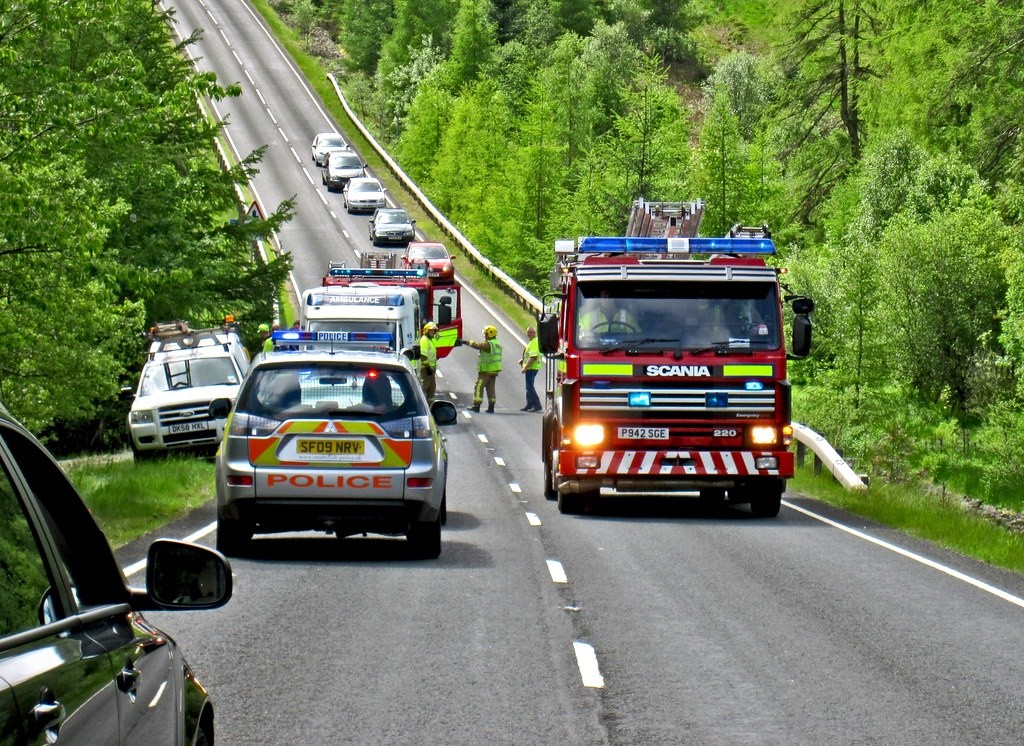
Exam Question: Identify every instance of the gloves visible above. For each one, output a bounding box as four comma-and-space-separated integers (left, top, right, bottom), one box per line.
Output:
458, 339, 468, 344
427, 368, 432, 375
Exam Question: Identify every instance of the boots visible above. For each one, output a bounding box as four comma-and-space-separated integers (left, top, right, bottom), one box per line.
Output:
485, 404, 494, 413
466, 404, 480, 413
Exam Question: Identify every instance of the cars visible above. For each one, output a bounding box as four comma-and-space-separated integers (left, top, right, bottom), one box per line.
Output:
343, 177, 387, 214
0, 400, 233, 746
310, 133, 350, 166
406, 243, 456, 285
369, 208, 416, 245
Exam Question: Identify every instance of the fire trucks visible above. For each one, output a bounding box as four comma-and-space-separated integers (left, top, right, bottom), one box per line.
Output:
297, 250, 463, 383
536, 198, 815, 519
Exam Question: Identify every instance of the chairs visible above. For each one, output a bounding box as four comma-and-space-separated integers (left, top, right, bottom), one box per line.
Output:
176, 560, 217, 597
362, 376, 392, 407
267, 376, 301, 409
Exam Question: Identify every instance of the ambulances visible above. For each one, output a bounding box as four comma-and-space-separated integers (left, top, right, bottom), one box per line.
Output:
300, 282, 420, 377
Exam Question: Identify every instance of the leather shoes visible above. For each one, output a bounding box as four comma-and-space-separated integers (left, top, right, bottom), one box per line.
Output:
520, 405, 542, 412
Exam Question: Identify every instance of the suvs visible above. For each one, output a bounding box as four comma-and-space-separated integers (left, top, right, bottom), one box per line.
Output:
321, 151, 368, 194
208, 330, 458, 562
125, 313, 252, 465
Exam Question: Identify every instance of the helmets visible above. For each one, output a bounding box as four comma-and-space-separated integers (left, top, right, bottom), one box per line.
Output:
482, 325, 498, 338
424, 322, 438, 333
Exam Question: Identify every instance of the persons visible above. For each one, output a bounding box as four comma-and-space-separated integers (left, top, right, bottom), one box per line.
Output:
579, 287, 642, 333
221, 318, 300, 352
458, 326, 502, 413
518, 327, 543, 413
711, 300, 762, 336
419, 321, 438, 407
